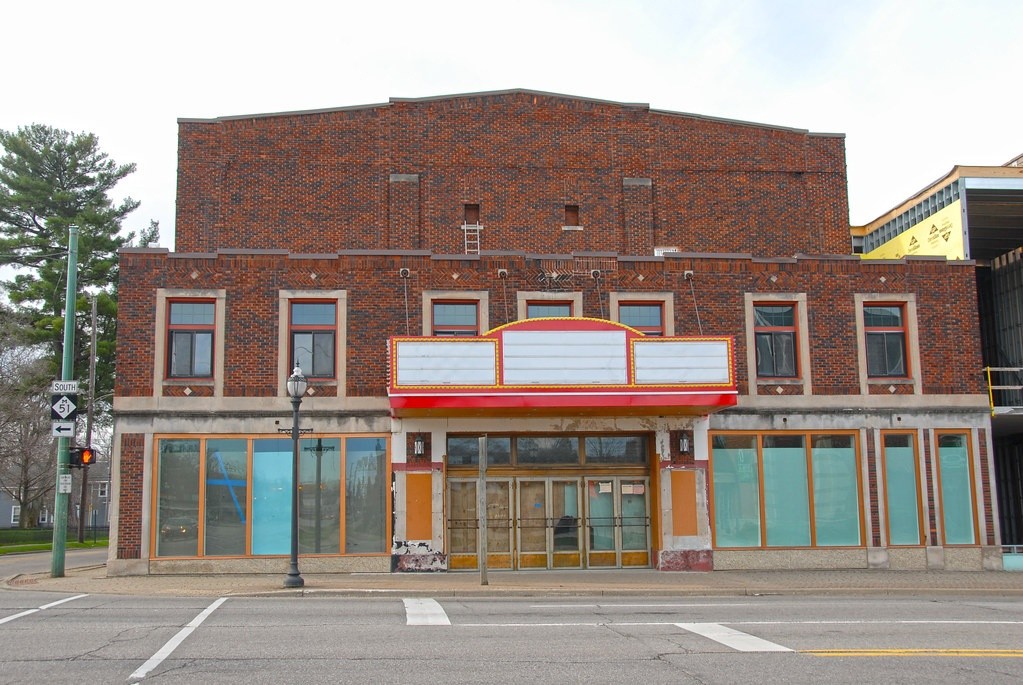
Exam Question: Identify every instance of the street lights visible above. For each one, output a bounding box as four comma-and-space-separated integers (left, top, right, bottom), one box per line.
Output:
284, 360, 307, 588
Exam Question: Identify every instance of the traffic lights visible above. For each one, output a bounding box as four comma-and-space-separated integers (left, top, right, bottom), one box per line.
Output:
69, 447, 97, 469
